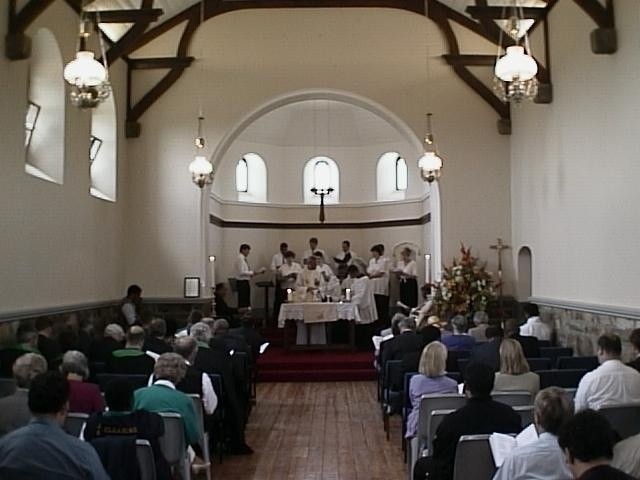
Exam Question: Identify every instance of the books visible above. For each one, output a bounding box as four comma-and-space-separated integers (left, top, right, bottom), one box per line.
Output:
486, 421, 541, 468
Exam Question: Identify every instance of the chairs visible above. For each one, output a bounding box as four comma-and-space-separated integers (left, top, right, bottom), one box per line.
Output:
373, 340, 640, 480
0, 332, 259, 480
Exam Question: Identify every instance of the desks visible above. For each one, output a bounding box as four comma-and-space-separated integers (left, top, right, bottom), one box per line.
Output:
277, 303, 363, 354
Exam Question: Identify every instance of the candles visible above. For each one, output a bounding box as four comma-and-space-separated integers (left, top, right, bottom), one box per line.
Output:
346, 289, 350, 300
287, 288, 293, 302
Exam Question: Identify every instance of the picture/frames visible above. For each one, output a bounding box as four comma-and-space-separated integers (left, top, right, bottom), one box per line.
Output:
183, 276, 201, 299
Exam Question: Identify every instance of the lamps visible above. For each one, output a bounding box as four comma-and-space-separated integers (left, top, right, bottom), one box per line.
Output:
495, 0, 539, 109
62, 1, 112, 114
187, 1, 215, 189
416, 0, 444, 188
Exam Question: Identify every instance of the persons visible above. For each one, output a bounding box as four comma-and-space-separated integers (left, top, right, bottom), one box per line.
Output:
233, 243, 265, 309
412, 361, 523, 480
371, 298, 639, 442
491, 385, 576, 480
117, 285, 143, 330
268, 237, 418, 349
1, 310, 267, 480
557, 402, 638, 480
611, 429, 640, 480
215, 283, 243, 316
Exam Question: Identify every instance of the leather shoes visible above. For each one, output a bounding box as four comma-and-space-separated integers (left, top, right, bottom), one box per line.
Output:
192, 462, 211, 474
